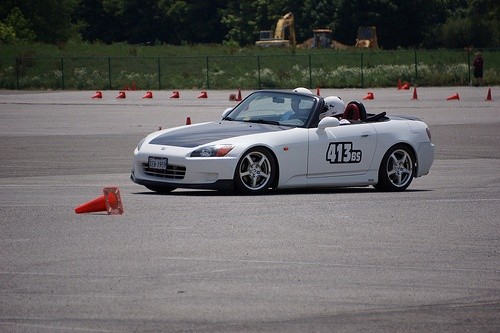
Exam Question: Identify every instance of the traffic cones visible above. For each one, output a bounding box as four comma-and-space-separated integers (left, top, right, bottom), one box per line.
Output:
159, 127, 161, 130
198, 92, 207, 98
398, 80, 402, 90
74, 186, 124, 215
238, 90, 242, 101
92, 91, 102, 98
185, 117, 191, 125
446, 93, 460, 100
363, 92, 374, 100
485, 88, 492, 101
116, 92, 125, 98
402, 82, 409, 90
170, 92, 179, 98
316, 88, 320, 96
143, 92, 152, 98
411, 87, 419, 100
230, 94, 238, 101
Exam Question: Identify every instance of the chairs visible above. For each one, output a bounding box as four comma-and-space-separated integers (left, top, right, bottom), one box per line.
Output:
342, 101, 367, 122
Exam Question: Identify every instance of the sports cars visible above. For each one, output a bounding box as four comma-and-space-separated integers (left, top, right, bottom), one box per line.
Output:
129, 90, 435, 195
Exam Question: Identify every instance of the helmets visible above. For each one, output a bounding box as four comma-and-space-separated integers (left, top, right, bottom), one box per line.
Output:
290, 87, 313, 113
318, 96, 346, 121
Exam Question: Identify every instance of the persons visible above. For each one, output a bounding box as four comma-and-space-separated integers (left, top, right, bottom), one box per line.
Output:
281, 87, 314, 122
318, 96, 349, 127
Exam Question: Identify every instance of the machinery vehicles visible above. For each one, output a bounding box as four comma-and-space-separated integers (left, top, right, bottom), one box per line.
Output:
304, 26, 378, 49
255, 12, 296, 47
